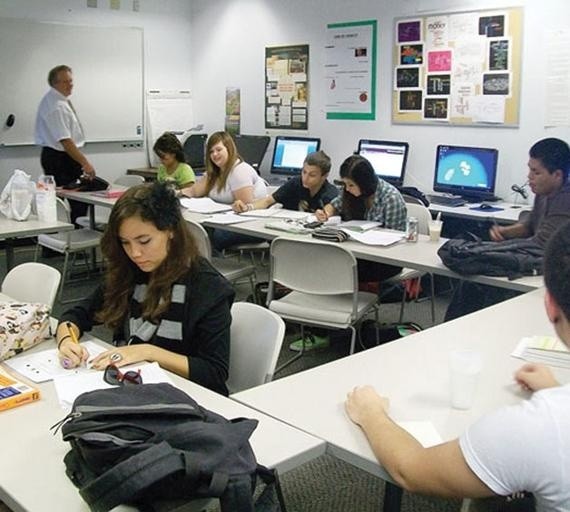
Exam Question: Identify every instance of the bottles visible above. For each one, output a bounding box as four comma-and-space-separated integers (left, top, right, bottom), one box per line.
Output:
36, 175, 57, 226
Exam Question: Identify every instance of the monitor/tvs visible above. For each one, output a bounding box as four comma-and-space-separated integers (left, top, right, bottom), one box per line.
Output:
270, 134, 322, 176
234, 135, 271, 176
433, 144, 499, 203
357, 139, 409, 188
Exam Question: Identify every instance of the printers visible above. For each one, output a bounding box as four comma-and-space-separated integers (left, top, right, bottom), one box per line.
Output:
154, 123, 208, 168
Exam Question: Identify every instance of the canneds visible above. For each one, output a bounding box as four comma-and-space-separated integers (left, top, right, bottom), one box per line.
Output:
406, 217, 418, 242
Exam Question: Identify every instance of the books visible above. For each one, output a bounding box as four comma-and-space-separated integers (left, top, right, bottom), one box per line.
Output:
179, 195, 385, 245
0, 363, 40, 412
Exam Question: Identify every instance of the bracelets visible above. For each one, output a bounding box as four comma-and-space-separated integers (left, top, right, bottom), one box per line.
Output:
57, 335, 71, 349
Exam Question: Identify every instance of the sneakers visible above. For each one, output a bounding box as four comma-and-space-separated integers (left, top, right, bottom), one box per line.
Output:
290, 332, 329, 351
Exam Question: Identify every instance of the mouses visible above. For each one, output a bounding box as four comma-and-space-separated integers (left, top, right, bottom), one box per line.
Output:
480, 204, 493, 209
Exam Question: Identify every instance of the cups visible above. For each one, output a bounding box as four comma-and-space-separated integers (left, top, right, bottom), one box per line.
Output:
428, 220, 444, 244
10, 182, 33, 221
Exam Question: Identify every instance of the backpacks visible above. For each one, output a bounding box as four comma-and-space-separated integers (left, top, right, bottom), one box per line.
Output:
437, 231, 546, 280
50, 383, 287, 512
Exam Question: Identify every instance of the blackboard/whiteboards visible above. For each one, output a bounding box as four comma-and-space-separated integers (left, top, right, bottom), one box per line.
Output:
0, 18, 144, 147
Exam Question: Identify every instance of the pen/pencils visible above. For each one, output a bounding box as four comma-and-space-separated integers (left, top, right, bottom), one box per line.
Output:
319, 199, 328, 222
66, 321, 87, 368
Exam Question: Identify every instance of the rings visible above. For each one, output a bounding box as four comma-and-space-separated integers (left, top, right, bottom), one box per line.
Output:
63, 358, 71, 367
110, 353, 122, 363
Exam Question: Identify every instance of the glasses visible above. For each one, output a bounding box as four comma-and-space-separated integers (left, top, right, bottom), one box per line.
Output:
104, 365, 142, 387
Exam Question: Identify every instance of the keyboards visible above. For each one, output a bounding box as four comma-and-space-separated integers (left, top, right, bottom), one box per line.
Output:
427, 195, 465, 208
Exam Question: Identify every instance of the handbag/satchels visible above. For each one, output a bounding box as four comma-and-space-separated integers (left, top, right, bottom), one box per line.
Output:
0, 302, 53, 363
354, 319, 423, 354
246, 281, 293, 309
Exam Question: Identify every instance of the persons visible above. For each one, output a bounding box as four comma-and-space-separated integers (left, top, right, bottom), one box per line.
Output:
36, 65, 96, 259
153, 131, 196, 189
443, 138, 569, 323
56, 180, 236, 397
343, 221, 570, 512
235, 150, 338, 304
177, 131, 268, 258
288, 153, 406, 353
